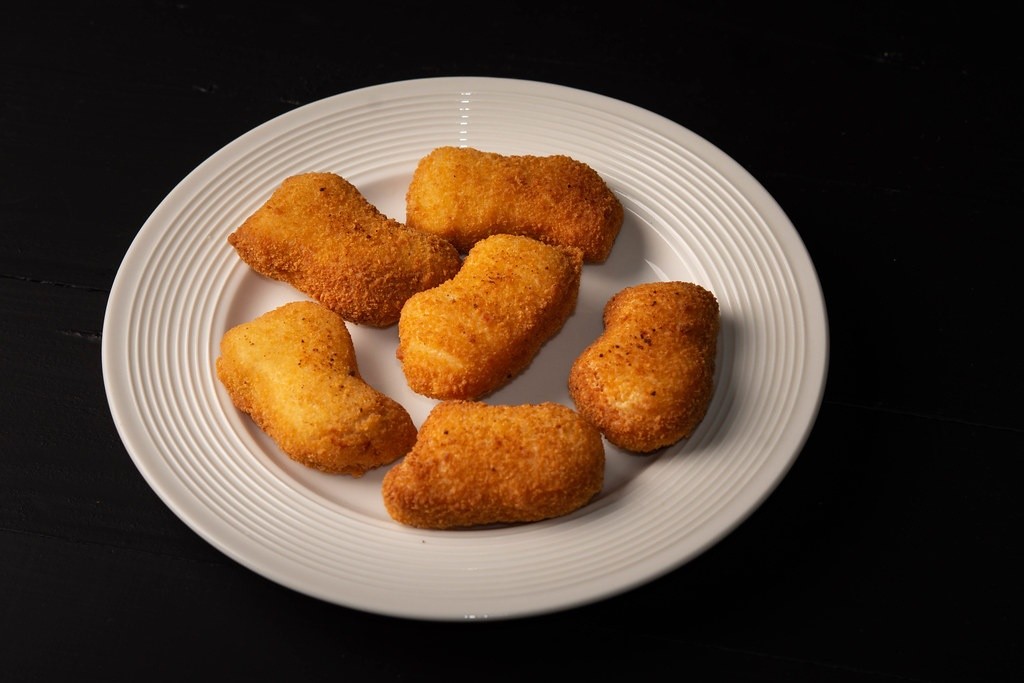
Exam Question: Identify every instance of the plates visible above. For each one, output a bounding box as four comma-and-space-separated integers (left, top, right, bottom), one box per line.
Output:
99, 75, 827, 623
